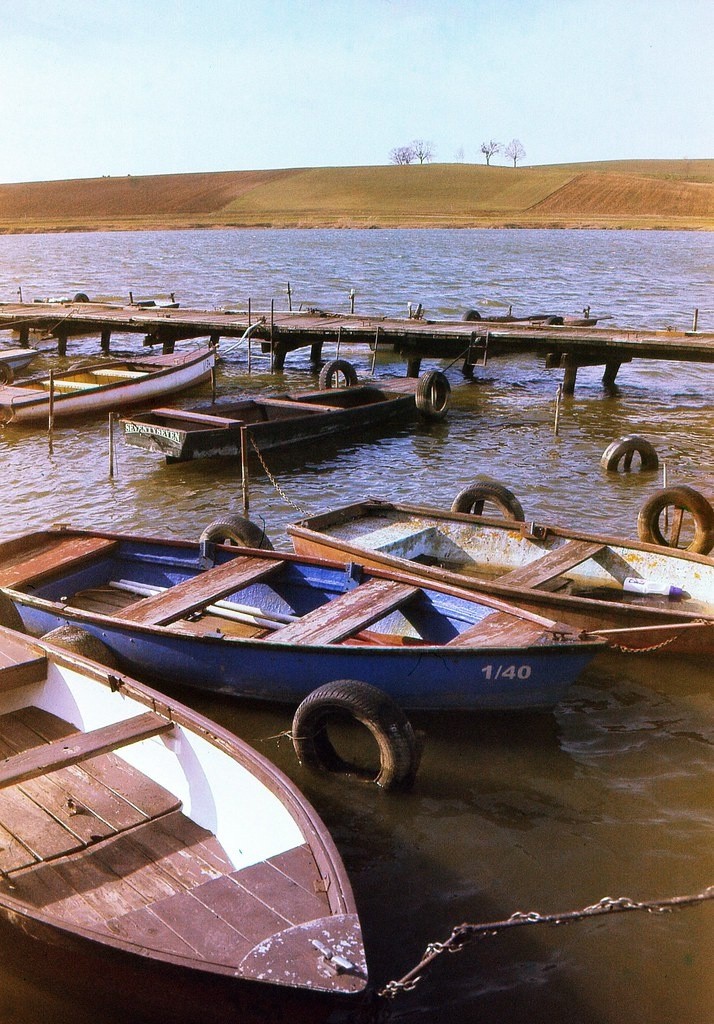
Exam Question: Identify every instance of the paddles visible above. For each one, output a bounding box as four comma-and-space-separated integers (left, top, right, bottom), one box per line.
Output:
119, 578, 441, 646
108, 581, 374, 646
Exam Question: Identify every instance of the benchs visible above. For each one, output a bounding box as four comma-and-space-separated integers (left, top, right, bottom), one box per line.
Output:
492, 538, 607, 588
446, 613, 547, 647
1, 635, 47, 691
41, 380, 100, 390
151, 407, 243, 428
262, 578, 421, 644
362, 378, 419, 400
89, 369, 148, 378
127, 352, 206, 371
108, 556, 286, 626
1, 712, 175, 789
0, 386, 62, 405
86, 843, 331, 968
0, 536, 120, 594
253, 397, 344, 413
319, 517, 438, 561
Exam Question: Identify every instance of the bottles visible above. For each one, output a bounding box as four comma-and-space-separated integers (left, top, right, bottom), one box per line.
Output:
623, 577, 682, 596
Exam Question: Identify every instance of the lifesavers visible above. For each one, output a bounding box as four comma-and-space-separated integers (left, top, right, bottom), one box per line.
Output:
319, 360, 358, 390
291, 681, 420, 791
73, 293, 90, 302
636, 486, 714, 555
415, 371, 451, 419
600, 435, 659, 471
0, 361, 14, 386
463, 310, 482, 322
545, 317, 564, 325
198, 516, 275, 552
451, 483, 524, 522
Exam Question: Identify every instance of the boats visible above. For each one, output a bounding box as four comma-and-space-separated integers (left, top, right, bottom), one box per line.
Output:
0, 521, 609, 714
0, 339, 220, 425
467, 311, 615, 326
117, 376, 429, 460
284, 495, 714, 655
0, 623, 372, 1009
32, 300, 180, 309
0, 343, 38, 375
